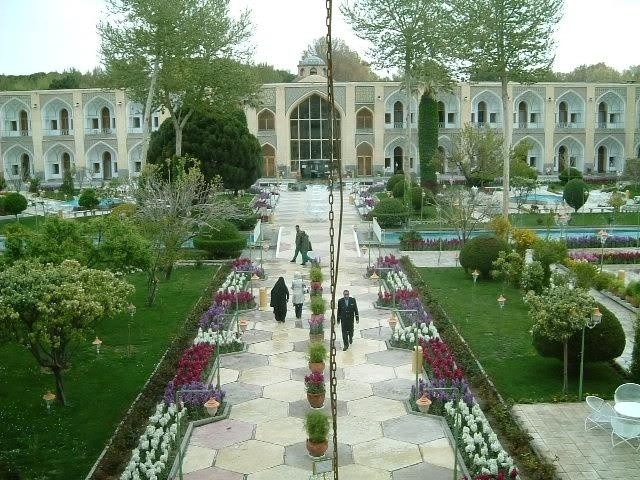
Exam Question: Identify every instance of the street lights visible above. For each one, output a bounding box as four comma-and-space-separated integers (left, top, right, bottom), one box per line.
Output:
421, 188, 425, 220
370, 268, 395, 307
217, 314, 247, 390
596, 230, 607, 271
572, 308, 602, 399
389, 310, 419, 394
233, 270, 260, 332
175, 389, 220, 480
415, 388, 460, 480
361, 241, 379, 266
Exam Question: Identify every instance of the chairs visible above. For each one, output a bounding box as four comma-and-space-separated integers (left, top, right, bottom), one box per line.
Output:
611, 417, 640, 453
585, 396, 616, 435
613, 383, 640, 403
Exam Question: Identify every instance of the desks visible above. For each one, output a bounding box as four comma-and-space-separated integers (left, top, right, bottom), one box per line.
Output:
614, 401, 640, 420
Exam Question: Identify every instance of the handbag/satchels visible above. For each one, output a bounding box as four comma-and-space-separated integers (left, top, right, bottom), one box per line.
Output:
301, 284, 309, 294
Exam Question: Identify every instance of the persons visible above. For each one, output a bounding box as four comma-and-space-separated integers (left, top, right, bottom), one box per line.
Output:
291, 271, 305, 318
270, 277, 289, 323
290, 224, 303, 262
300, 231, 314, 265
386, 165, 391, 176
337, 290, 359, 351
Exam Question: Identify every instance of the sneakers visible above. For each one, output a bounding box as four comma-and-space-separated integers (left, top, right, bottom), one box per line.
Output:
300, 262, 305, 265
343, 339, 353, 352
289, 259, 296, 263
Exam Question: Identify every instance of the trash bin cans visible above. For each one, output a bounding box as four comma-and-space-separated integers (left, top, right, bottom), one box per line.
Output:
258, 287, 269, 310
412, 346, 424, 375
